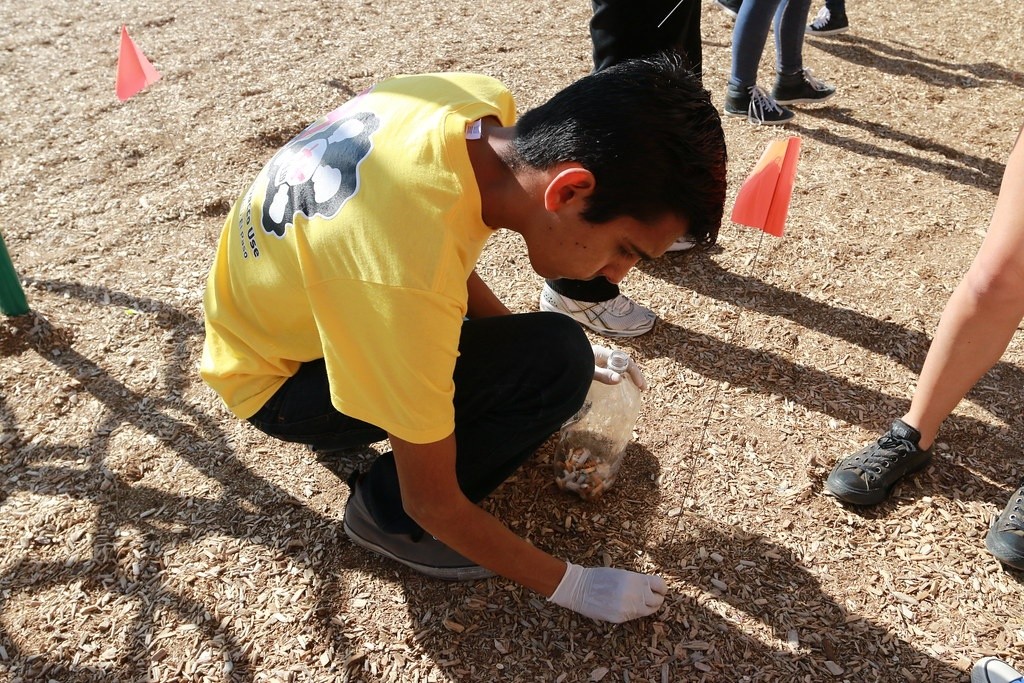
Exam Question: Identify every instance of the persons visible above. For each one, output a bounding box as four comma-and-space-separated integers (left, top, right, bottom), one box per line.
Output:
538, 0, 704, 337
197, 50, 728, 624
723, 0, 836, 126
821, 124, 1024, 576
712, 0, 850, 36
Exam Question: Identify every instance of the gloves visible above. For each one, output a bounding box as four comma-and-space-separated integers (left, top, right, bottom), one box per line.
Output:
546, 562, 667, 624
591, 344, 648, 391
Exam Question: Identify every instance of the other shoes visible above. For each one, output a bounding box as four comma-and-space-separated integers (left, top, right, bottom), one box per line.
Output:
805, 13, 849, 35
343, 469, 500, 580
971, 655, 1024, 683
716, 0, 742, 18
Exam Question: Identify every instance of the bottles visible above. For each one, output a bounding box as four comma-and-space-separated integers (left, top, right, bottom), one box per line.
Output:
551, 350, 643, 500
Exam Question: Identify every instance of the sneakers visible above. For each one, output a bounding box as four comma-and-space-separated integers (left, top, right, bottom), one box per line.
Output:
827, 417, 933, 505
987, 476, 1024, 571
725, 88, 795, 124
771, 71, 836, 106
539, 283, 657, 339
666, 236, 695, 251
